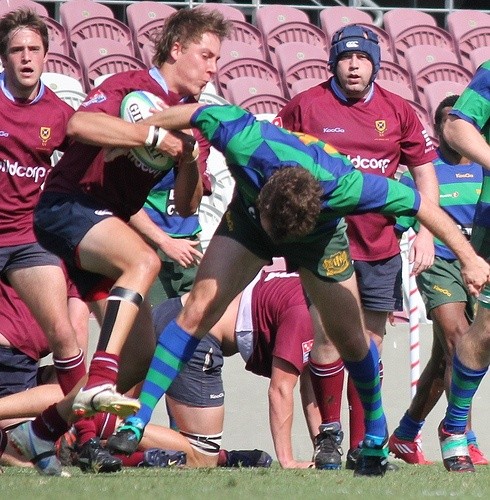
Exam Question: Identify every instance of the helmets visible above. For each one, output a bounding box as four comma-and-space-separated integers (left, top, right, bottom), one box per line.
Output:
327, 25, 380, 87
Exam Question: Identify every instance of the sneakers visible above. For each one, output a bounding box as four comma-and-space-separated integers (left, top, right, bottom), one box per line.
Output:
386, 432, 434, 466
345, 448, 399, 473
0, 419, 188, 475
72, 383, 141, 419
465, 429, 489, 466
438, 418, 475, 473
354, 432, 389, 477
313, 422, 343, 470
223, 449, 272, 468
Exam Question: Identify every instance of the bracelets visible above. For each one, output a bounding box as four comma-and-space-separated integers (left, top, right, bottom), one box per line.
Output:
145, 126, 168, 149
151, 125, 159, 148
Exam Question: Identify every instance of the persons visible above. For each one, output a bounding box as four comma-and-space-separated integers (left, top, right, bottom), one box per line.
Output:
0, 6, 490, 478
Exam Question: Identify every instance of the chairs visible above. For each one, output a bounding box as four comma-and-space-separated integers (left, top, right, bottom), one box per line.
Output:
0, 0, 490, 177
